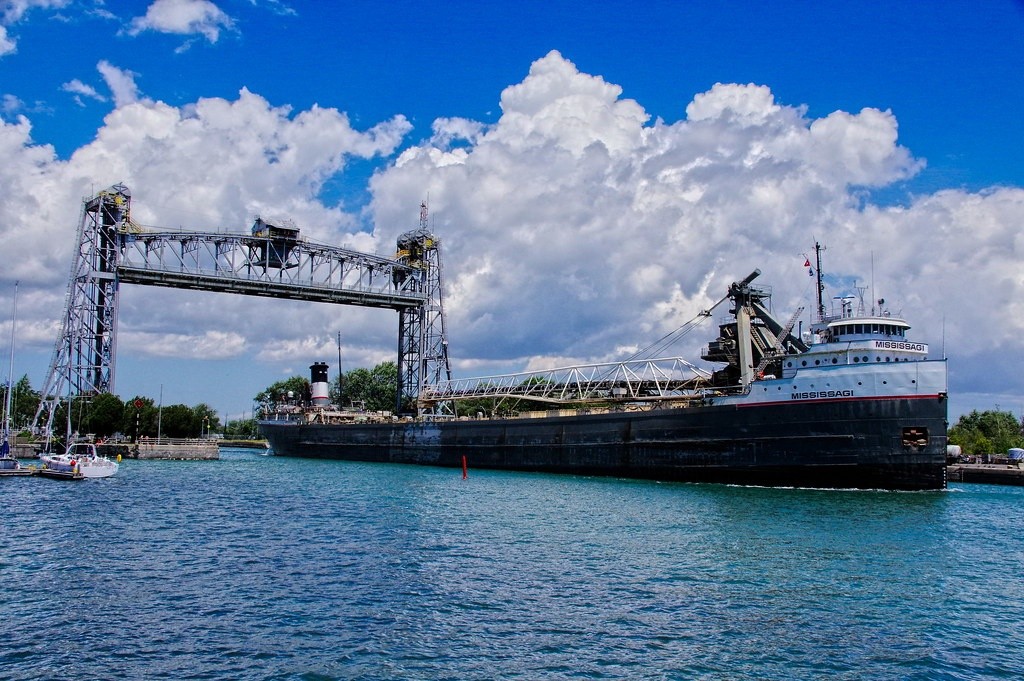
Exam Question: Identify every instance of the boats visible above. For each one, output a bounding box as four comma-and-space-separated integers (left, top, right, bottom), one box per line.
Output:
39, 436, 120, 482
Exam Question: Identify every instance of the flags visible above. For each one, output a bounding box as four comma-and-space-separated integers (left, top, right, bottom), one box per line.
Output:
800, 259, 815, 277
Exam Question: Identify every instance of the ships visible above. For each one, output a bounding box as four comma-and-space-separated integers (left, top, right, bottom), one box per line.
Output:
259, 237, 952, 495
